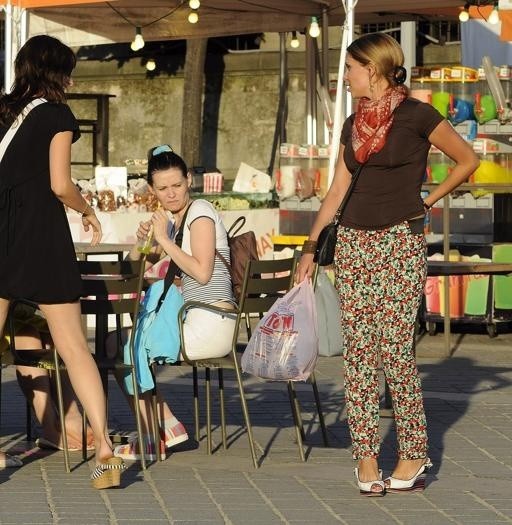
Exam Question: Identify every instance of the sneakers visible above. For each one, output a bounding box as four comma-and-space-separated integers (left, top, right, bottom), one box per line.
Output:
0, 454, 23, 467
113, 440, 167, 461
127, 422, 189, 449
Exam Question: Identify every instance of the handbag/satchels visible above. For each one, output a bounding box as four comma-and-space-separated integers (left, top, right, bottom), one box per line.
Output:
312, 223, 338, 266
311, 272, 344, 358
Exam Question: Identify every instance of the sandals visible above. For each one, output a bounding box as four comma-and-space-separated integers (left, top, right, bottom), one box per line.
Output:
354, 468, 385, 497
383, 457, 435, 494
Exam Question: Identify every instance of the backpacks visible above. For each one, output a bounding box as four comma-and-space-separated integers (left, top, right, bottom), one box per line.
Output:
188, 216, 262, 298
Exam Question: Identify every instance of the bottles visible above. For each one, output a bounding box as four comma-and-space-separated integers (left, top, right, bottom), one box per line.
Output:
136, 225, 154, 253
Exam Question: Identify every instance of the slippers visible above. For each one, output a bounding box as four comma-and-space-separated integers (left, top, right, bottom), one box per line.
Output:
35, 438, 96, 451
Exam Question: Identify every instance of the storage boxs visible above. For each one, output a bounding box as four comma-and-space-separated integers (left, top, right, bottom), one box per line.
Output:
278, 198, 333, 235
430, 191, 496, 236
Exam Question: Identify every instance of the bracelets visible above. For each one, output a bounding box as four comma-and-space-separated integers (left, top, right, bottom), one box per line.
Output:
420, 200, 433, 212
79, 200, 89, 218
299, 240, 320, 259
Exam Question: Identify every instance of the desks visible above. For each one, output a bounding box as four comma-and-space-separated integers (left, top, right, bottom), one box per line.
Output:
60, 204, 279, 327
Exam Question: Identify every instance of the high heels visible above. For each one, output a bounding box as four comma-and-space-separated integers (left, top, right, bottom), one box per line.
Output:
90, 457, 129, 490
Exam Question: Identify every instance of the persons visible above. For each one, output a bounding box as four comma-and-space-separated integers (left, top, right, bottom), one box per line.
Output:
102, 142, 235, 462
1, 32, 123, 491
1, 301, 99, 470
292, 34, 479, 497
